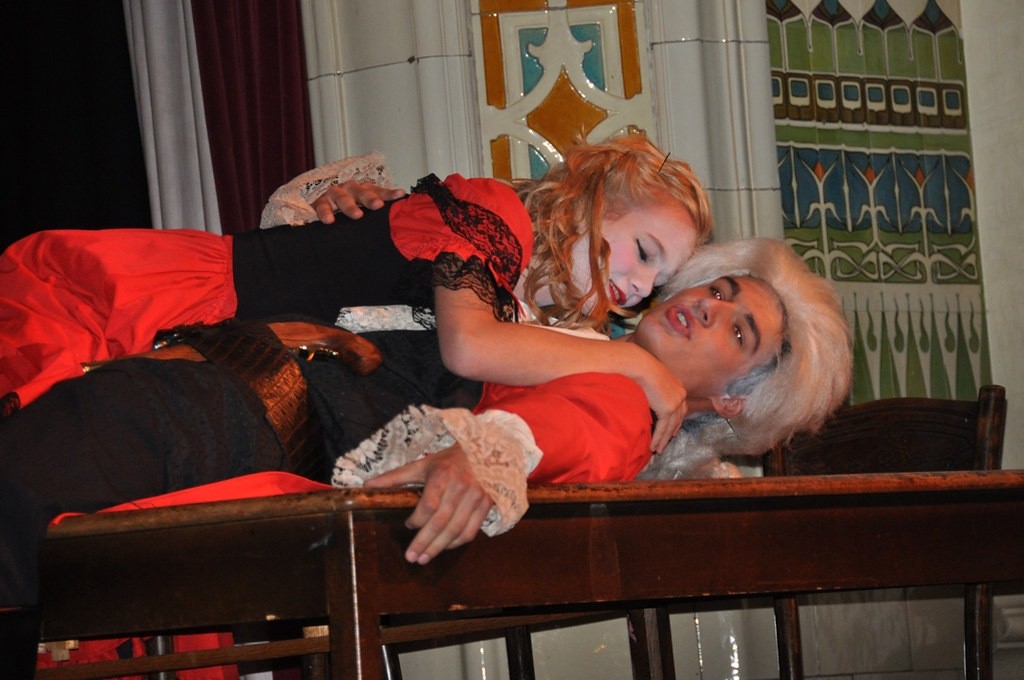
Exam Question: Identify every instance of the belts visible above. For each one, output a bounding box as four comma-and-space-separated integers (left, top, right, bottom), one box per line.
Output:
152, 320, 309, 478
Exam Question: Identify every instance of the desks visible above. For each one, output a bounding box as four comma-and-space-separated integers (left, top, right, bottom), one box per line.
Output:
0, 464, 1024, 680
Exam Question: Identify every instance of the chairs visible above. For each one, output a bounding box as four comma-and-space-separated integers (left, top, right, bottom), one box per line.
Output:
763, 384, 1008, 680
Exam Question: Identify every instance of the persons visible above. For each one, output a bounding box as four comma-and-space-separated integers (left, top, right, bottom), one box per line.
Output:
0, 149, 853, 680
1, 128, 714, 456
